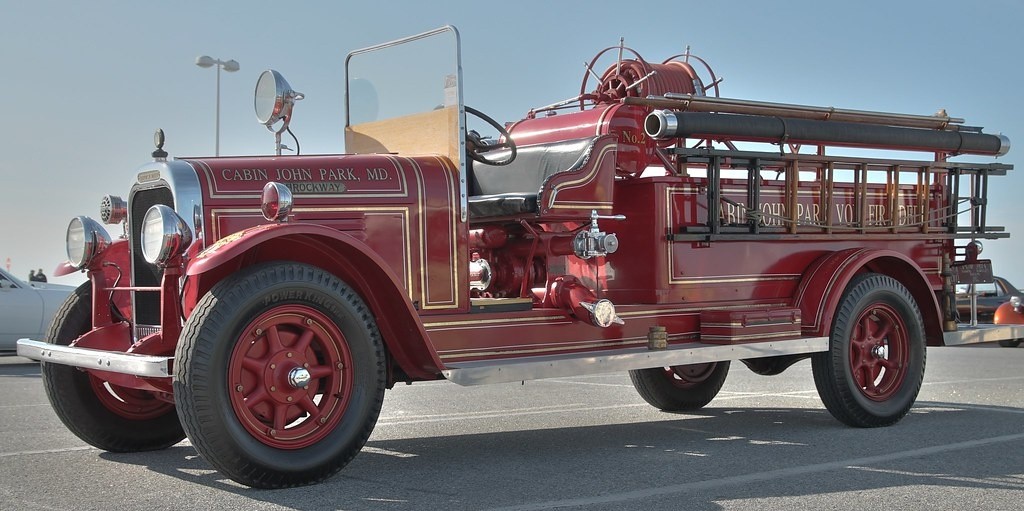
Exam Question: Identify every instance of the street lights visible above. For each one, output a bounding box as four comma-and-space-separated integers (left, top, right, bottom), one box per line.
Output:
195, 55, 242, 155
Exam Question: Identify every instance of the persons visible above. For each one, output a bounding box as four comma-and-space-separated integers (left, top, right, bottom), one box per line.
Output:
29, 268, 47, 283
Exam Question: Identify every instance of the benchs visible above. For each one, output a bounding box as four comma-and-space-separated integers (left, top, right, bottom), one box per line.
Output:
469, 132, 619, 221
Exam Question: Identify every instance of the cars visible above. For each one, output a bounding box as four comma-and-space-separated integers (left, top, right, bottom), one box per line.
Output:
0, 267, 78, 359
955, 275, 1024, 322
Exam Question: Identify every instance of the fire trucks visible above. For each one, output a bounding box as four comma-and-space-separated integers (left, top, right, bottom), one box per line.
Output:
14, 23, 1010, 492
